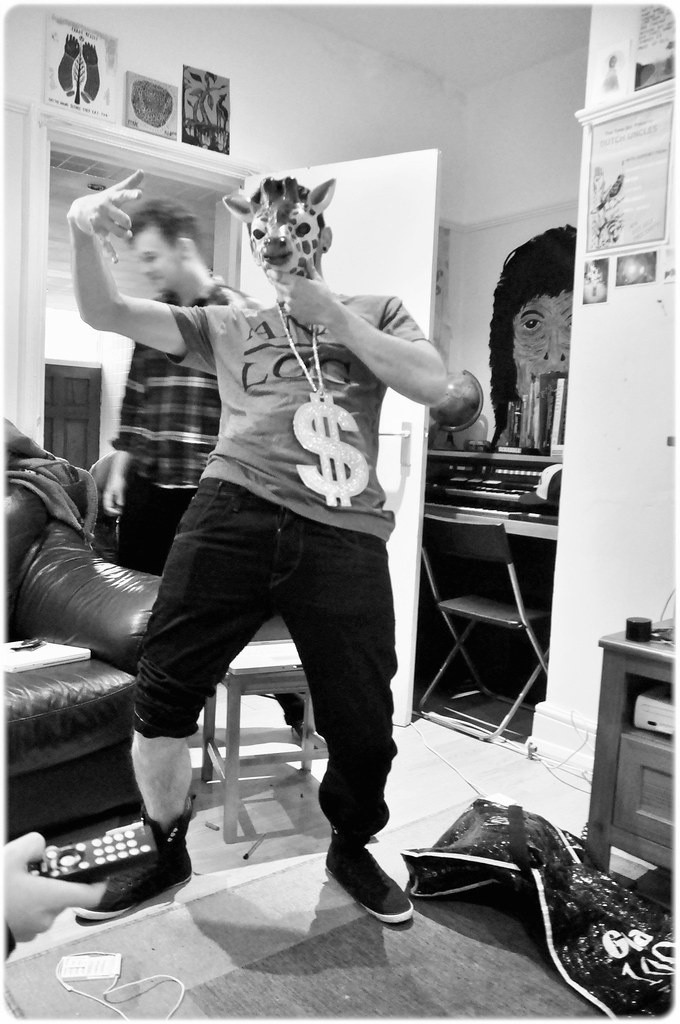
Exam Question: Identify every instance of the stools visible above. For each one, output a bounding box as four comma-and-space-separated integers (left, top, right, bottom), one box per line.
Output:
201, 639, 330, 845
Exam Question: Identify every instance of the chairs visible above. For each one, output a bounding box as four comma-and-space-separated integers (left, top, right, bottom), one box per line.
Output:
418, 513, 551, 739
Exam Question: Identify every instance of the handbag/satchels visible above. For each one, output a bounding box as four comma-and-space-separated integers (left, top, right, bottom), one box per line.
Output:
404, 801, 672, 1018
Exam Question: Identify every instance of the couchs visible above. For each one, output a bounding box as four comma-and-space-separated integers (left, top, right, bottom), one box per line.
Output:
4, 463, 164, 839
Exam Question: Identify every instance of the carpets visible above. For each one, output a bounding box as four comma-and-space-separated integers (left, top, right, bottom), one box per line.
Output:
3, 804, 651, 1021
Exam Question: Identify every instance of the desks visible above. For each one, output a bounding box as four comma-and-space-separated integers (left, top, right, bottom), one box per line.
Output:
584, 619, 674, 872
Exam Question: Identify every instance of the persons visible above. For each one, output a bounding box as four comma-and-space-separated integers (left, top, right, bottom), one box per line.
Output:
102, 199, 257, 576
66, 168, 446, 924
4, 831, 106, 965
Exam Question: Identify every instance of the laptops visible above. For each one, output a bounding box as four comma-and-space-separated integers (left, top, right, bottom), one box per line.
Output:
2, 641, 92, 674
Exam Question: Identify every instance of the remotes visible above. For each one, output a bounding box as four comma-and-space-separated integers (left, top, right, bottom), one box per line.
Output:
26, 825, 159, 883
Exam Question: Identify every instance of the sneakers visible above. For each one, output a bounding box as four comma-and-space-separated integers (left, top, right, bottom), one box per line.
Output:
69, 795, 195, 919
326, 842, 407, 924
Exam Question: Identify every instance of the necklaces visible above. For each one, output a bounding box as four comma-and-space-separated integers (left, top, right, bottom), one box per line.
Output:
276, 302, 369, 507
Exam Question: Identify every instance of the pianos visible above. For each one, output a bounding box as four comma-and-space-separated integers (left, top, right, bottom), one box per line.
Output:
422, 465, 576, 513
